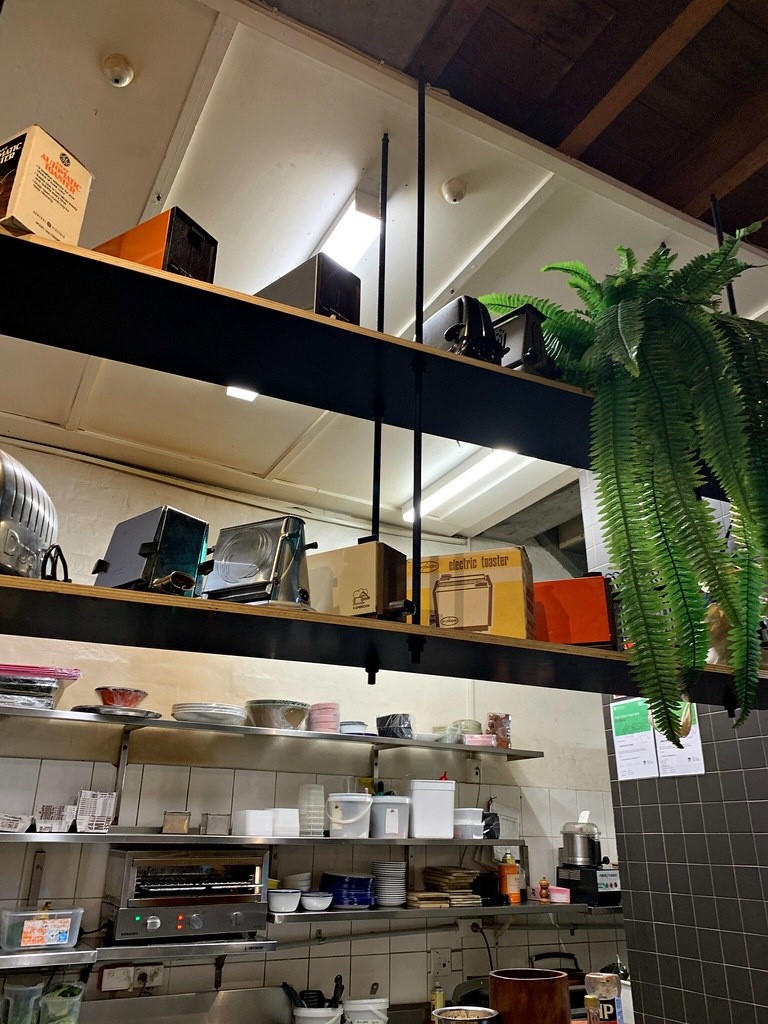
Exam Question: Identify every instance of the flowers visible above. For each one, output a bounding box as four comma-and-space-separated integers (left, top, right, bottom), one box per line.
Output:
463, 759, 483, 783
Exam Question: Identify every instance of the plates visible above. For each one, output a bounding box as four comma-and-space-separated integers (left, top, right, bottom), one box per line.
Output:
34, 804, 76, 833
371, 861, 407, 908
0, 663, 80, 709
70, 705, 162, 719
0, 815, 34, 833
170, 702, 247, 724
412, 733, 444, 741
76, 790, 118, 833
324, 873, 376, 909
452, 720, 483, 743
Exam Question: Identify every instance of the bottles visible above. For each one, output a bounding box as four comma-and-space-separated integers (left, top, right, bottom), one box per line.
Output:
584, 995, 602, 1024
499, 849, 521, 903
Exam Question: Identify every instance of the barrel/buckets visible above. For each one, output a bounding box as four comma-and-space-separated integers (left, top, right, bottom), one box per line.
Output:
403, 779, 456, 839
488, 967, 572, 1024
371, 796, 411, 839
293, 1008, 343, 1024
325, 793, 373, 839
344, 998, 391, 1024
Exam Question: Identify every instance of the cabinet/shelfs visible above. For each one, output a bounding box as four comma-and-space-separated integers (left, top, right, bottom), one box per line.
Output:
0, 705, 623, 970
0, 0, 768, 710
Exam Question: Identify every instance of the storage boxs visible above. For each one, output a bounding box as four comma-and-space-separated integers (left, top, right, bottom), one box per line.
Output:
201, 814, 230, 836
161, 810, 191, 834
407, 546, 535, 640
0, 123, 94, 246
535, 886, 571, 904
402, 778, 455, 840
0, 907, 85, 952
232, 808, 300, 837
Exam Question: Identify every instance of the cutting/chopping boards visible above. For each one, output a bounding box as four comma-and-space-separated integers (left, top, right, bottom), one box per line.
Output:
407, 866, 482, 908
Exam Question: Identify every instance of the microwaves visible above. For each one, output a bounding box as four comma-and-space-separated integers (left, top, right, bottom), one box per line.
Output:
101, 846, 269, 941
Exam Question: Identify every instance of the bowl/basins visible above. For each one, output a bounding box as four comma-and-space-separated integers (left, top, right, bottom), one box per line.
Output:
95, 686, 149, 707
284, 872, 311, 891
301, 892, 332, 909
268, 878, 300, 915
245, 699, 311, 729
432, 1005, 499, 1024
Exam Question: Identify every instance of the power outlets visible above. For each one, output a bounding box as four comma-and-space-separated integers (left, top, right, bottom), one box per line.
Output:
101, 964, 165, 992
457, 919, 483, 937
431, 948, 451, 978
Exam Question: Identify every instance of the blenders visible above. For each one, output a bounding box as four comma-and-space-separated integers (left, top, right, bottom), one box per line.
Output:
556, 820, 622, 906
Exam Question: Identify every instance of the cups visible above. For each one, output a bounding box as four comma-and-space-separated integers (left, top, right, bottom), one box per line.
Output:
297, 784, 326, 838
